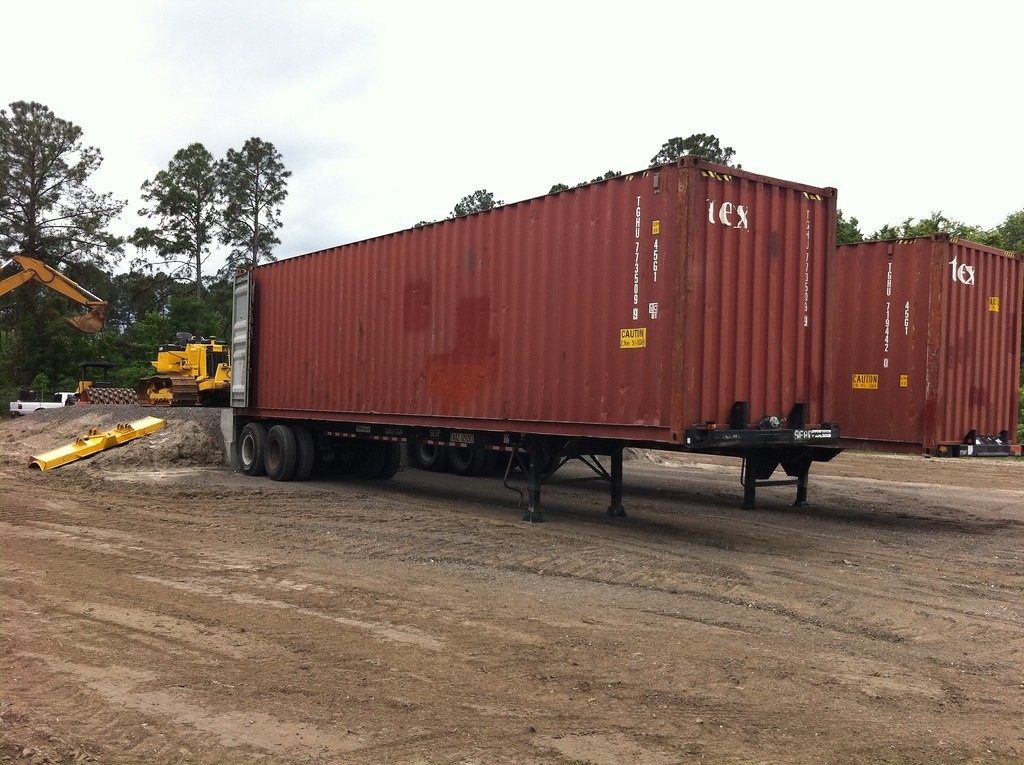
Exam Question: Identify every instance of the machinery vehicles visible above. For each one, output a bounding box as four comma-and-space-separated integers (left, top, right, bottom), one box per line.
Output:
65, 362, 138, 406
0, 253, 110, 334
134, 332, 231, 408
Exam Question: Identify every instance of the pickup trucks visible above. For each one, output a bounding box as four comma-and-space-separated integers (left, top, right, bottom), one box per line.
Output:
9, 392, 77, 417
